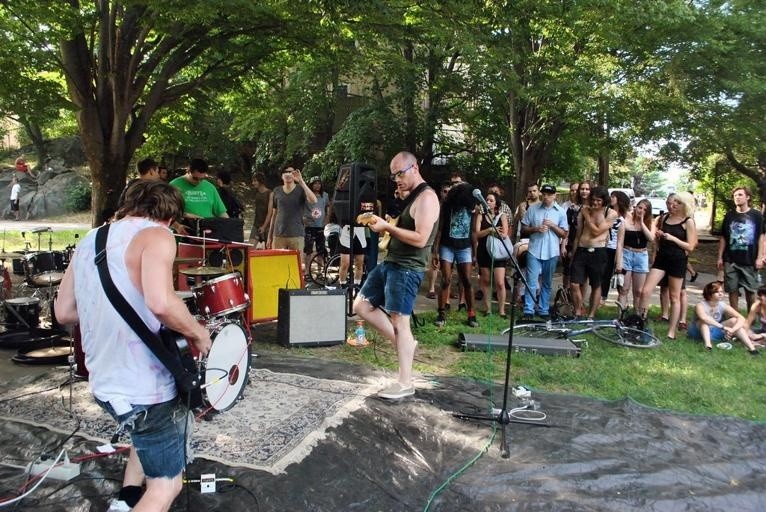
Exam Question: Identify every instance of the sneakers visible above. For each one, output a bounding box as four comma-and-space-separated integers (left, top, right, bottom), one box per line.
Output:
426, 291, 438, 299
378, 384, 416, 398
652, 313, 667, 323
690, 270, 699, 282
105, 498, 130, 512
435, 312, 447, 326
467, 315, 478, 326
678, 320, 686, 332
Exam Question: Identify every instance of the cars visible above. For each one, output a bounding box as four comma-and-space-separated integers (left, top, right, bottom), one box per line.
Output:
637, 198, 667, 218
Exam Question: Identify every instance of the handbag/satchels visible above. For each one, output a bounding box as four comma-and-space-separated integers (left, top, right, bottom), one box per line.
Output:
486, 214, 513, 260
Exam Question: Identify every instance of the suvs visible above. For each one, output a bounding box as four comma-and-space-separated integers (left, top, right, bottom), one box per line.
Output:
607, 188, 636, 204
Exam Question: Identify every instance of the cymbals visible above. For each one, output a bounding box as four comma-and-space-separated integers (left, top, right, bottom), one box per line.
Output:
180, 264, 230, 276
25, 346, 74, 358
0, 251, 26, 260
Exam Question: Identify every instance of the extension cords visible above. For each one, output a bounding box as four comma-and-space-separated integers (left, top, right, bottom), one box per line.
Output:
23, 456, 82, 481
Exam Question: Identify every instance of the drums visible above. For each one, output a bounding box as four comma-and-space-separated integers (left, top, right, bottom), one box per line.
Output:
190, 273, 249, 318
174, 314, 253, 420
26, 250, 66, 285
5, 297, 41, 328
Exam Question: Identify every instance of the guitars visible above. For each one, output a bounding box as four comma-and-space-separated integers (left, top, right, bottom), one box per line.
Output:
356, 212, 400, 251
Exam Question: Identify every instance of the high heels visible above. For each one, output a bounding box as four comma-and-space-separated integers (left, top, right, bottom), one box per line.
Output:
456, 303, 466, 311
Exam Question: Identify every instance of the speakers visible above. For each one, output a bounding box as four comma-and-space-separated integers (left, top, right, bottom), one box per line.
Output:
330, 162, 377, 226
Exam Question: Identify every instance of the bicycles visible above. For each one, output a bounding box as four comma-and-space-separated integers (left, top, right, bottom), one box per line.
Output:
502, 300, 661, 349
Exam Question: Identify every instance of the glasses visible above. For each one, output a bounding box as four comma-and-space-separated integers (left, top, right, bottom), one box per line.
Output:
451, 180, 460, 184
390, 165, 415, 179
188, 173, 204, 182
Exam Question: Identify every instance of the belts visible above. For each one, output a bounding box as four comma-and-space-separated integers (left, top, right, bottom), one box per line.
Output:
578, 246, 608, 254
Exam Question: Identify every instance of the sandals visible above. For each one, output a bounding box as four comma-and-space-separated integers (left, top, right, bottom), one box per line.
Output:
303, 273, 311, 282
451, 293, 459, 299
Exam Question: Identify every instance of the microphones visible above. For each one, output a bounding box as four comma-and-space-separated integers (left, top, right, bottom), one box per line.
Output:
472, 188, 491, 210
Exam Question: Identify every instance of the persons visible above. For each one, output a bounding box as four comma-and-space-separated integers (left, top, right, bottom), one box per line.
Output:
512, 183, 543, 309
566, 182, 589, 311
266, 164, 318, 265
247, 172, 273, 250
54, 180, 211, 512
474, 182, 513, 318
742, 284, 766, 346
301, 176, 331, 281
159, 167, 167, 181
9, 178, 21, 221
431, 175, 479, 327
568, 186, 616, 325
338, 188, 375, 294
559, 182, 579, 305
716, 187, 766, 325
15, 154, 37, 180
215, 170, 243, 219
692, 281, 763, 357
125, 159, 203, 221
657, 215, 698, 282
615, 198, 656, 319
654, 193, 689, 330
640, 195, 698, 341
520, 185, 569, 320
353, 152, 440, 400
168, 159, 229, 237
601, 191, 630, 308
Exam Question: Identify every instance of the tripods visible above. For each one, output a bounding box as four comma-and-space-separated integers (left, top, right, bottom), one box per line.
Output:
453, 211, 556, 458
341, 225, 361, 317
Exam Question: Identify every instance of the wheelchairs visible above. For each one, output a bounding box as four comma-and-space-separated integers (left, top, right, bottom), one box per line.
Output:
310, 232, 352, 289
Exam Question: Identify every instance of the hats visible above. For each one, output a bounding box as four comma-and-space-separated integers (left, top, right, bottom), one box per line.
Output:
541, 185, 557, 194
308, 175, 319, 183
